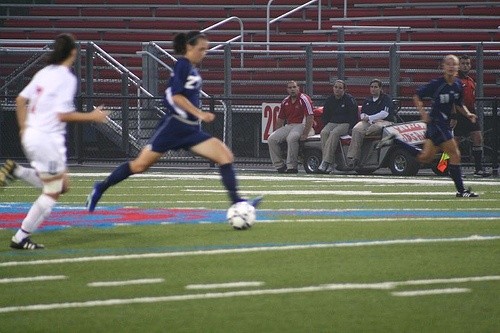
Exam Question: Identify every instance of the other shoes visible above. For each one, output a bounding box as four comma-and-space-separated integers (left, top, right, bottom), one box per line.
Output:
240, 195, 262, 207
473, 170, 491, 177
326, 163, 333, 171
85, 182, 102, 212
318, 160, 328, 172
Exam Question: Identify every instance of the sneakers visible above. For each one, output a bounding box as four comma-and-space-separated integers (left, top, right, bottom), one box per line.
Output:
0, 159, 18, 187
456, 190, 480, 199
10, 235, 44, 250
374, 135, 396, 150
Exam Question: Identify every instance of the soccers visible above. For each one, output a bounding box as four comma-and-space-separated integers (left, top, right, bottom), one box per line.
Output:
227, 202, 256, 230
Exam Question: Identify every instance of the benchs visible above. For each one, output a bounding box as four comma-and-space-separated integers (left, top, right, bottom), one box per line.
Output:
0, 0, 500, 111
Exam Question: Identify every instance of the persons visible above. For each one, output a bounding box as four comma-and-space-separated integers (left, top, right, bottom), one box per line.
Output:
267, 80, 314, 173
87, 30, 264, 213
346, 80, 396, 171
0, 33, 111, 249
449, 56, 490, 179
318, 80, 359, 174
392, 54, 479, 197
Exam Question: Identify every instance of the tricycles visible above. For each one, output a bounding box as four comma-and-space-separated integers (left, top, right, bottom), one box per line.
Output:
280, 99, 439, 175
